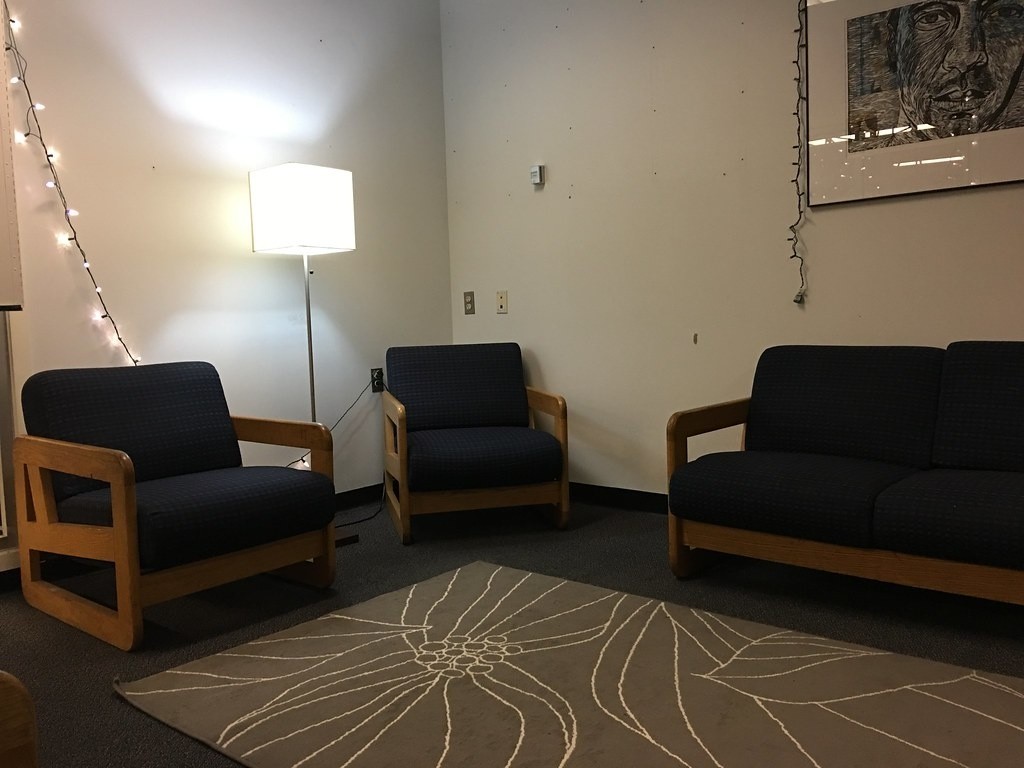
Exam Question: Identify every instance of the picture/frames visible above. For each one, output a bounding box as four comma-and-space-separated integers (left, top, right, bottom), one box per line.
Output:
803, 1, 1024, 206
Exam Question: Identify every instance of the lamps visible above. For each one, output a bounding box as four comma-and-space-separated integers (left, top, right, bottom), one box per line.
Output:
245, 161, 361, 542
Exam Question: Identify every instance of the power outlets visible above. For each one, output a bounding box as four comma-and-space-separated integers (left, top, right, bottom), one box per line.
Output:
371, 368, 384, 393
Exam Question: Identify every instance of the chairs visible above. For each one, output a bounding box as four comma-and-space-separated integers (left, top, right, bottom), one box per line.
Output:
12, 362, 338, 653
377, 341, 572, 542
664, 337, 1024, 607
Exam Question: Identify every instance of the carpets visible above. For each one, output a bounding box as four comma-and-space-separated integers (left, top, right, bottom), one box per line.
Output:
110, 557, 1024, 767
